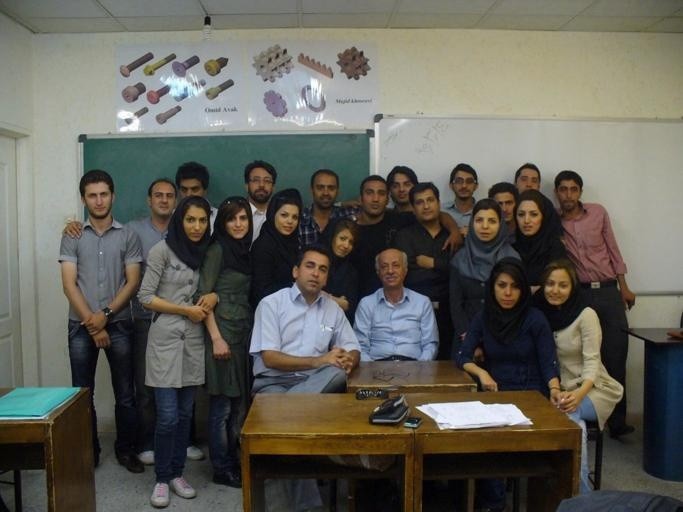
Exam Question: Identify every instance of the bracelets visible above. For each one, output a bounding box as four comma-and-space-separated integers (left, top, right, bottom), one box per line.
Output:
102, 307, 114, 322
550, 385, 560, 390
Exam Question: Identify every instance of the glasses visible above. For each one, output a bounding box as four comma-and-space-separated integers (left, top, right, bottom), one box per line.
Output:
355, 387, 390, 400
249, 176, 274, 185
452, 178, 477, 184
223, 198, 246, 205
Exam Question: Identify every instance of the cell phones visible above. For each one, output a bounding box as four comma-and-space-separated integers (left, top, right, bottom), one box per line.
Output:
404, 417, 423, 428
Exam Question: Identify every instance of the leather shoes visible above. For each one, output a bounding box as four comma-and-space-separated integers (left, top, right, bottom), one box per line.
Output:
610, 425, 635, 439
234, 465, 242, 480
116, 454, 144, 473
213, 472, 242, 488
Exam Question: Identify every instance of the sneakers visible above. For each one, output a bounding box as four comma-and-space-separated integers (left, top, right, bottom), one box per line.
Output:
137, 450, 155, 465
187, 445, 205, 460
169, 475, 196, 498
150, 483, 169, 507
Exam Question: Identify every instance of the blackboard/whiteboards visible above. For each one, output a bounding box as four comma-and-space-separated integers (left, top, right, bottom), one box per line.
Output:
77, 130, 375, 226
373, 114, 683, 295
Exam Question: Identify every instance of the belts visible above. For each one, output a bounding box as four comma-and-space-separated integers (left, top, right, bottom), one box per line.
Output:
578, 279, 617, 289
375, 354, 417, 361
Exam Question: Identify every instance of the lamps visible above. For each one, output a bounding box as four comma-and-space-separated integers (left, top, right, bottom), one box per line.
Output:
200, 15, 213, 41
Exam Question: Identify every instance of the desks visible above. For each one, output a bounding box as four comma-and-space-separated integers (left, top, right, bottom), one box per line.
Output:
0, 387, 96, 512
387, 389, 585, 512
347, 359, 474, 512
621, 324, 682, 482
239, 391, 415, 512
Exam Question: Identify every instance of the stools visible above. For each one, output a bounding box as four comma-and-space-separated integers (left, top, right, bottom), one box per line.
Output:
510, 424, 603, 512
1, 467, 26, 512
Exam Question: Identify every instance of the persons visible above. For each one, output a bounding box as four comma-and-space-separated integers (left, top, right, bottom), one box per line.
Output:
388, 182, 456, 361
137, 193, 221, 509
459, 256, 564, 512
440, 161, 482, 239
487, 182, 521, 245
351, 246, 440, 511
448, 196, 523, 360
512, 162, 562, 241
246, 249, 363, 512
535, 256, 625, 494
172, 161, 220, 236
243, 160, 361, 253
297, 167, 359, 250
553, 167, 638, 440
57, 170, 146, 475
313, 213, 362, 327
355, 173, 464, 299
193, 194, 259, 489
386, 165, 419, 239
509, 189, 557, 296
248, 186, 304, 313
60, 177, 206, 465
